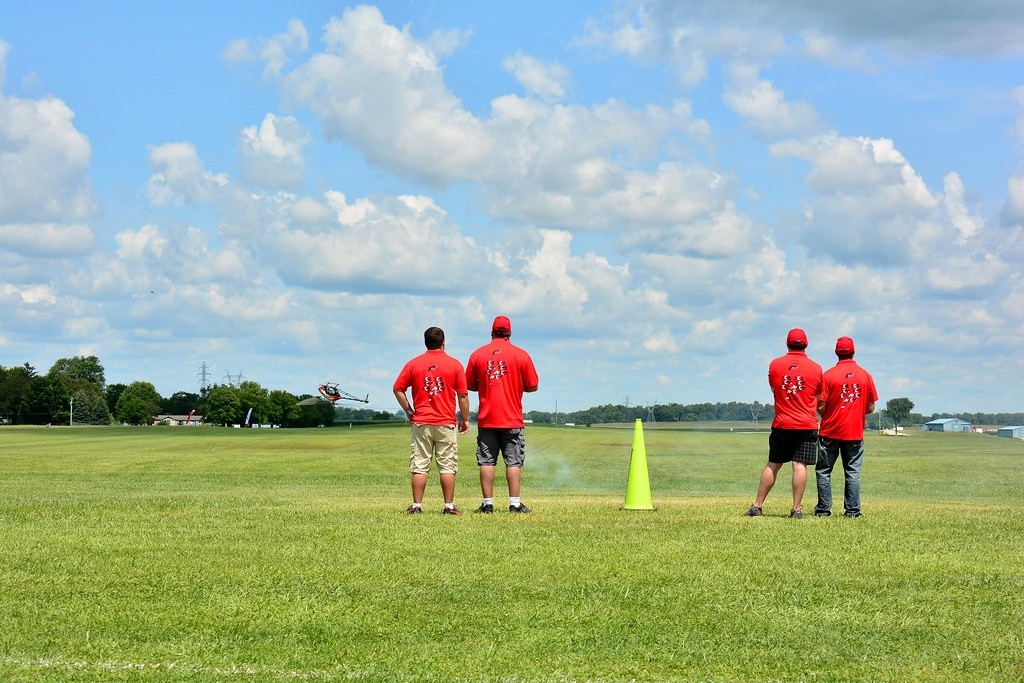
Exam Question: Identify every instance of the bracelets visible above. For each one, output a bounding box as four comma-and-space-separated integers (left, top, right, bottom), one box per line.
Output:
405, 408, 412, 414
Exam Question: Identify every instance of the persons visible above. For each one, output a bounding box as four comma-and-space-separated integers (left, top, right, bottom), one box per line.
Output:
393, 327, 469, 516
465, 315, 539, 513
743, 328, 823, 519
812, 336, 878, 518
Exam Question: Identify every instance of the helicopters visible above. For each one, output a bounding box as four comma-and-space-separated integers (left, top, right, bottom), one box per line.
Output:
318, 382, 371, 406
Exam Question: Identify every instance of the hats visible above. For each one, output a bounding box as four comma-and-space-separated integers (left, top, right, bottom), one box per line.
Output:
787, 328, 807, 345
493, 316, 511, 332
836, 336, 854, 354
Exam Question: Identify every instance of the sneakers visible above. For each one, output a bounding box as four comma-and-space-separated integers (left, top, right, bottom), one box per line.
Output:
444, 506, 461, 515
473, 503, 493, 513
509, 502, 532, 513
406, 505, 421, 513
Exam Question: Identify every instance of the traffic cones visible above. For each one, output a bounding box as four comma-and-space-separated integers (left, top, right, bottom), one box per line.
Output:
617, 419, 657, 512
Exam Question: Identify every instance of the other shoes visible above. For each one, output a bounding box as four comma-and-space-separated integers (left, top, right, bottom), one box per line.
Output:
744, 504, 762, 516
790, 510, 802, 519
